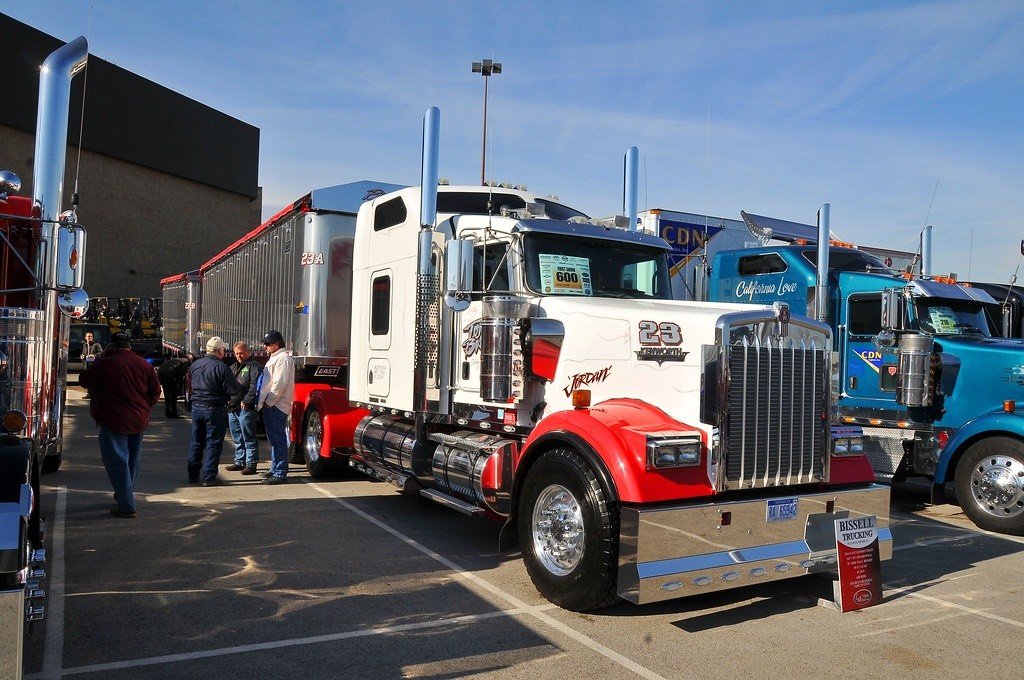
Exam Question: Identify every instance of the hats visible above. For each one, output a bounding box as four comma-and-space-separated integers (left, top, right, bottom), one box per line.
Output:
111, 332, 129, 341
206, 337, 228, 352
260, 330, 282, 343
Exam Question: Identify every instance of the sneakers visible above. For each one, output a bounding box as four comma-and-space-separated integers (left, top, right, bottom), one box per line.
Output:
259, 471, 288, 485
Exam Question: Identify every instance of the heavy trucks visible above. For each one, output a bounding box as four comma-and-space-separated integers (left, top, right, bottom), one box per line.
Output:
157, 100, 897, 614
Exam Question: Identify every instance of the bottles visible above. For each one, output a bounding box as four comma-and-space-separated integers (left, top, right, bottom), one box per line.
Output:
82, 359, 85, 366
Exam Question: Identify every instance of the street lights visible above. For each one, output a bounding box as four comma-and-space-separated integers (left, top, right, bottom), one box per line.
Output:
471, 57, 502, 188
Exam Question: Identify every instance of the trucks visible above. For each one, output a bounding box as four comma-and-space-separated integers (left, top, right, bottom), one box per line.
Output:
0, 34, 103, 677
676, 201, 1024, 536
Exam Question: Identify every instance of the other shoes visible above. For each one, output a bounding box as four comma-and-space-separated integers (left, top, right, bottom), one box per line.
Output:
82, 394, 92, 399
203, 478, 224, 487
111, 506, 137, 518
189, 477, 201, 484
241, 468, 256, 475
225, 465, 245, 471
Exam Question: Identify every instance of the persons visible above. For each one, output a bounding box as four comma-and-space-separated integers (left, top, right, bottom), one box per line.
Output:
159, 355, 193, 419
79, 331, 161, 519
187, 330, 295, 487
